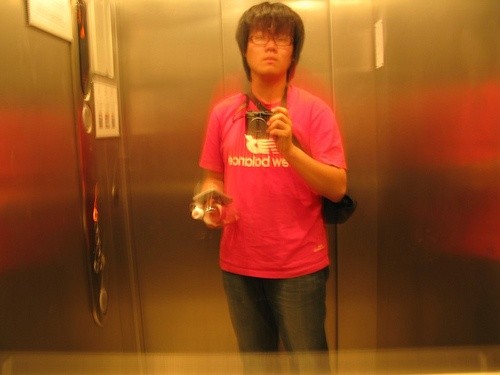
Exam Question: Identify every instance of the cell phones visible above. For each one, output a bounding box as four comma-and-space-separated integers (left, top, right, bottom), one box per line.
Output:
193, 189, 232, 206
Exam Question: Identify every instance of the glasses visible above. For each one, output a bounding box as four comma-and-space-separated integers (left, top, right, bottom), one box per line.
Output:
247, 35, 294, 45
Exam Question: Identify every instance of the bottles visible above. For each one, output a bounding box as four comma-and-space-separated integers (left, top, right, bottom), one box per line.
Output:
191, 203, 241, 224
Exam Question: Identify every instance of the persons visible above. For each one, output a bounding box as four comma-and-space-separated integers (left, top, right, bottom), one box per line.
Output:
198, 2, 348, 375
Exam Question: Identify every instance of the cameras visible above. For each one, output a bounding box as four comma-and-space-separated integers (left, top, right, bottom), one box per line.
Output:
245, 111, 273, 139
